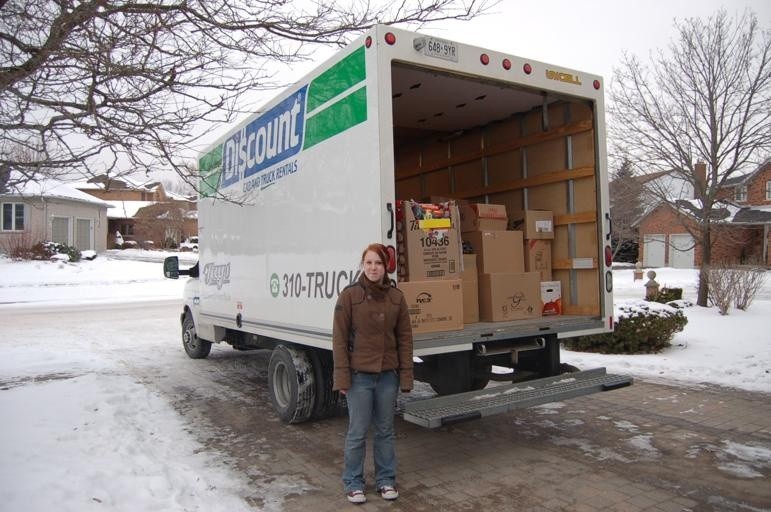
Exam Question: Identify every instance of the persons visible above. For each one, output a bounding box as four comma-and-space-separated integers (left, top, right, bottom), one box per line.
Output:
332, 243, 414, 502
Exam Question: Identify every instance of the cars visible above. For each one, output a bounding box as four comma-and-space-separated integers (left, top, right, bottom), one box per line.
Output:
180, 236, 199, 252
610, 238, 640, 264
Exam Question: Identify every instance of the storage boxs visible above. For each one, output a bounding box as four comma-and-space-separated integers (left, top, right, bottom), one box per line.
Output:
397, 195, 563, 332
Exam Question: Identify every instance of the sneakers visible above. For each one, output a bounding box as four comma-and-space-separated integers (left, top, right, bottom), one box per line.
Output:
346, 488, 367, 503
379, 483, 400, 500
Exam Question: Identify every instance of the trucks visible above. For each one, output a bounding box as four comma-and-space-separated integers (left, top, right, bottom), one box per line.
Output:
164, 22, 633, 430
